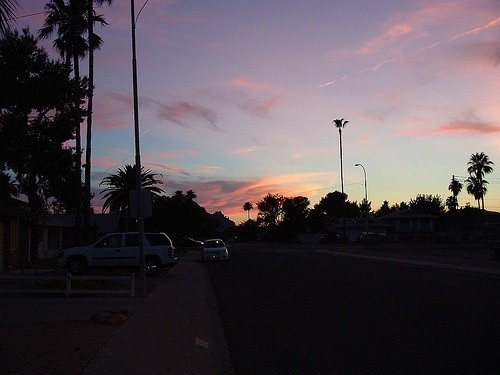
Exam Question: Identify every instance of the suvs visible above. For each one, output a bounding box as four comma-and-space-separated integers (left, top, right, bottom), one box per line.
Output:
55, 232, 178, 275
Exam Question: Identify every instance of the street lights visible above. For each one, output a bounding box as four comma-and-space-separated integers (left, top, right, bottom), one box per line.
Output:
355, 164, 368, 247
333, 118, 348, 240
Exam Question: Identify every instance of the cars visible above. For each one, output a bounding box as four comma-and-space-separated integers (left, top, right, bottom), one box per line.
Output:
201, 239, 228, 261
320, 232, 350, 244
173, 235, 204, 248
356, 231, 393, 244
